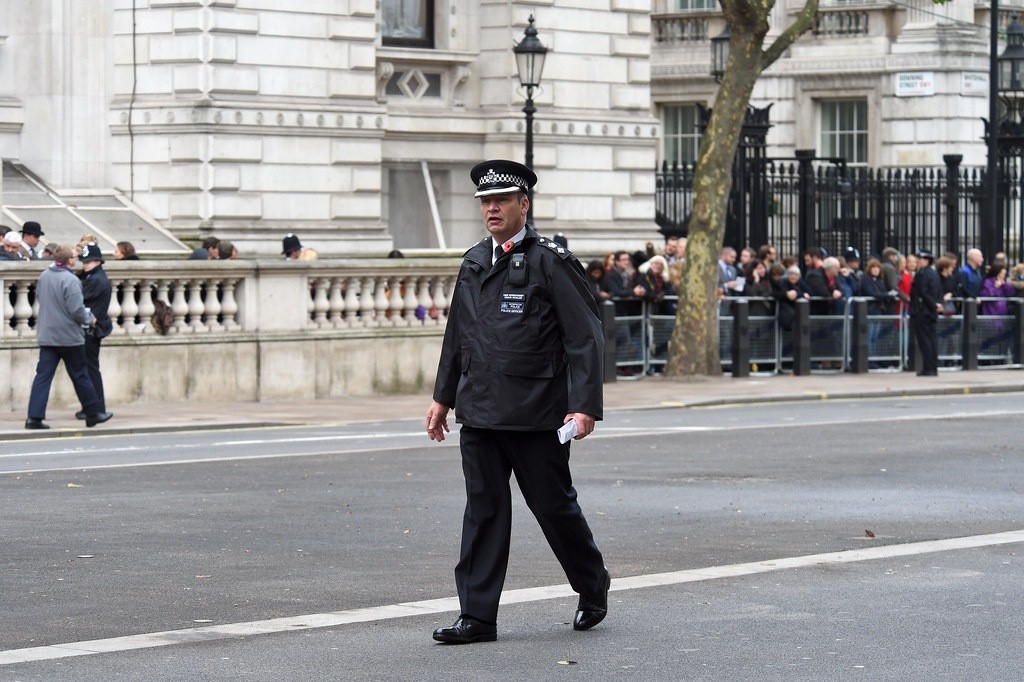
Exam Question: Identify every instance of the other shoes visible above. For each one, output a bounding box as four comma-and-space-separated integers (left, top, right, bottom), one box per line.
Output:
76, 410, 86, 419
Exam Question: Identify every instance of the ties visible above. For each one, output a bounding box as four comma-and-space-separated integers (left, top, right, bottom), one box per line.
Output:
30, 248, 35, 256
495, 245, 503, 260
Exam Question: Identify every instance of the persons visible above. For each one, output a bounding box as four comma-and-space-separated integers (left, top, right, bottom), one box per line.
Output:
385, 250, 406, 320
0, 221, 99, 327
281, 237, 319, 299
188, 239, 238, 321
587, 236, 1024, 376
113, 241, 142, 324
908, 253, 944, 376
424, 159, 611, 644
25, 243, 114, 430
73, 243, 113, 420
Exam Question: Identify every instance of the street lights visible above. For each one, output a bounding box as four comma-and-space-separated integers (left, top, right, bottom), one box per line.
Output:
512, 14, 549, 233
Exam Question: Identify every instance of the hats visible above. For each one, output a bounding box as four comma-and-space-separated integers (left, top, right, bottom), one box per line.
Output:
918, 249, 933, 265
78, 242, 106, 264
470, 160, 538, 199
280, 234, 303, 255
845, 247, 861, 261
18, 222, 44, 235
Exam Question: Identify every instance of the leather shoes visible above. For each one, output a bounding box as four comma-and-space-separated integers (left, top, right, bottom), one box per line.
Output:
86, 412, 112, 427
25, 417, 50, 429
433, 614, 497, 644
574, 565, 611, 631
